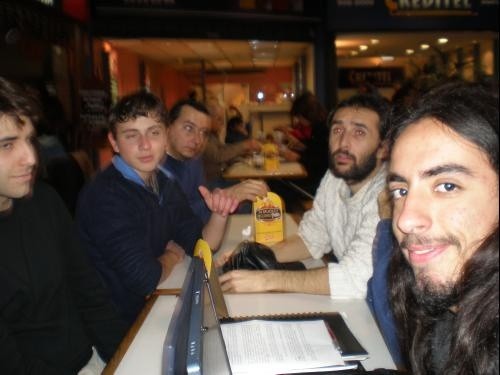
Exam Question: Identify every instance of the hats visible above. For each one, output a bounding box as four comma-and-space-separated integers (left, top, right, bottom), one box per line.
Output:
222, 239, 307, 274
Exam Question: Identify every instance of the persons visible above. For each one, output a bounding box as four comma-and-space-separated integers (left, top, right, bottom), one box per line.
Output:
75, 91, 235, 326
226, 114, 253, 144
356, 64, 500, 111
0, 78, 119, 375
276, 92, 336, 201
201, 102, 261, 187
186, 89, 204, 113
383, 82, 500, 375
215, 92, 389, 299
161, 98, 269, 222
19, 65, 67, 145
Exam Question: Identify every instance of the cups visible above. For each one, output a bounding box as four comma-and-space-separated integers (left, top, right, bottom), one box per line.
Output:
252, 151, 263, 169
239, 153, 252, 172
264, 142, 280, 172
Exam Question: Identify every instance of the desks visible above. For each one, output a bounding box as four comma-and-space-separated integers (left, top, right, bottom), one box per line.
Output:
222, 143, 316, 204
95, 210, 382, 375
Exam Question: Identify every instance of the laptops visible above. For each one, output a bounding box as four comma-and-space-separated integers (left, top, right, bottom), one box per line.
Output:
187, 258, 232, 375
162, 256, 199, 375
